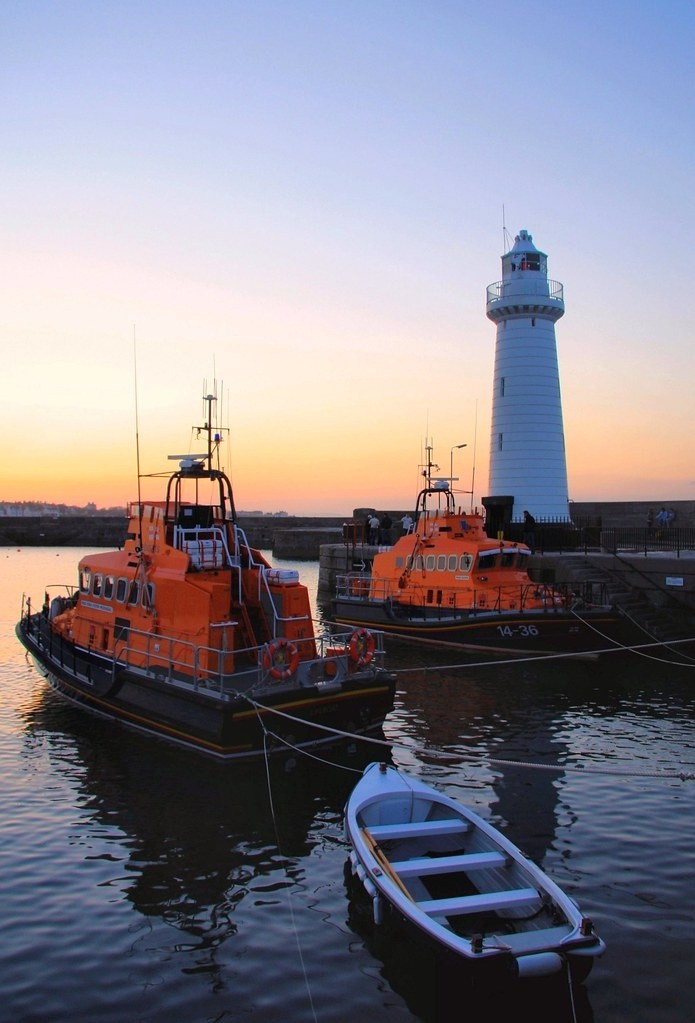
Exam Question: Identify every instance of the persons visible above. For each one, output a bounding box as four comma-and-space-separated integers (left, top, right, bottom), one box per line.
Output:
364, 513, 392, 545
522, 510, 536, 555
646, 508, 654, 537
656, 508, 676, 529
394, 513, 413, 536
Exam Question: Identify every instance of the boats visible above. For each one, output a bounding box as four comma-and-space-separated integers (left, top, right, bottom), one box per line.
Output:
342, 760, 607, 982
331, 398, 626, 666
13, 322, 396, 777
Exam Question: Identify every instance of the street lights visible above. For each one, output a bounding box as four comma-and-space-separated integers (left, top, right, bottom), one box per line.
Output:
450, 443, 468, 513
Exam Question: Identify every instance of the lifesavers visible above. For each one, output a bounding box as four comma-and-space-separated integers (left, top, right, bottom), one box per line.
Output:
264, 639, 298, 679
349, 629, 375, 666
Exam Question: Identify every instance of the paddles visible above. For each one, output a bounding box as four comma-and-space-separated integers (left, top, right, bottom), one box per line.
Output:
356, 811, 417, 906
